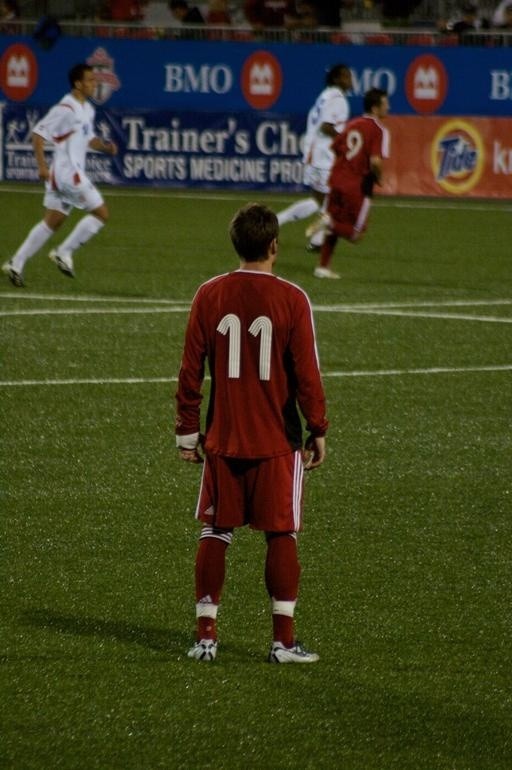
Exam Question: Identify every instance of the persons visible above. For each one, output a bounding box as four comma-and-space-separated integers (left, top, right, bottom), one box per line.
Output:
175, 204, 329, 664
1, 64, 119, 287
303, 86, 392, 279
272, 63, 352, 254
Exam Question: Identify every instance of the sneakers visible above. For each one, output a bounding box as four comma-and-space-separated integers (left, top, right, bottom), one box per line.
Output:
268, 640, 320, 664
47, 246, 77, 279
304, 211, 341, 279
187, 637, 219, 663
0, 258, 32, 288
